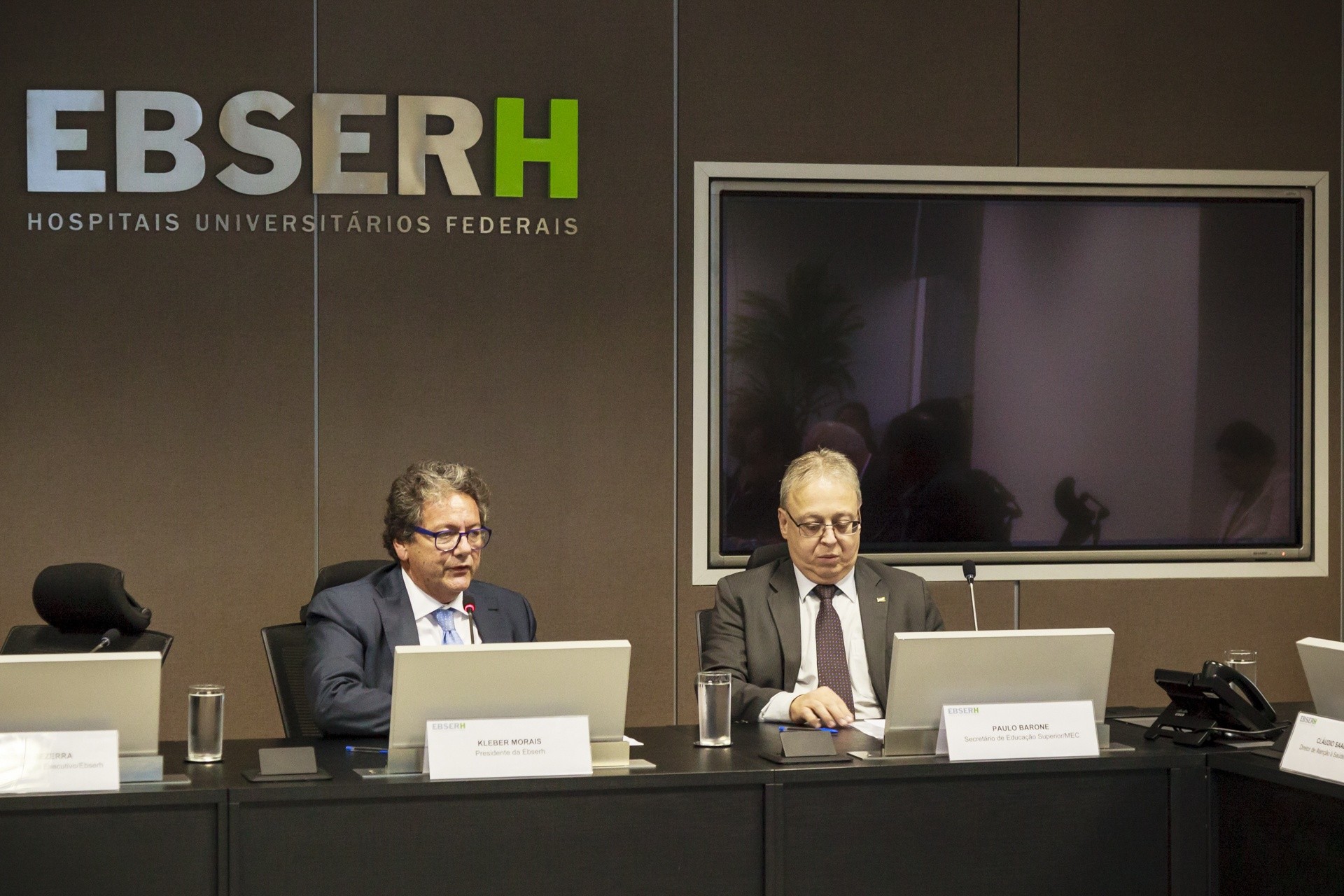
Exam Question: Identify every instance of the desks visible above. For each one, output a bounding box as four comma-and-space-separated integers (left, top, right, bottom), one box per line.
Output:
0, 703, 1344, 896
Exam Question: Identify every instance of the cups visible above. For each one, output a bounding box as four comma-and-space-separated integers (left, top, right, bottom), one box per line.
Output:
186, 684, 225, 760
1225, 650, 1257, 707
697, 671, 732, 745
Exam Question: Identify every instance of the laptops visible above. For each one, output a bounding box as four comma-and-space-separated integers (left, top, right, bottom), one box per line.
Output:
387, 640, 632, 776
847, 628, 1116, 754
1297, 637, 1344, 719
0, 652, 161, 755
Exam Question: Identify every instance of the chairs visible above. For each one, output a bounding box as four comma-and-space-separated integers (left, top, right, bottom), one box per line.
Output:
0, 563, 173, 669
261, 560, 393, 738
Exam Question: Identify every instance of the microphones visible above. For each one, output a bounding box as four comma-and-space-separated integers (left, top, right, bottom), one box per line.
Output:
963, 560, 980, 632
464, 594, 477, 645
90, 630, 120, 653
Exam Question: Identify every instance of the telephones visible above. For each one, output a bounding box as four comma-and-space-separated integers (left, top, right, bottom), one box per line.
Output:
1142, 660, 1282, 746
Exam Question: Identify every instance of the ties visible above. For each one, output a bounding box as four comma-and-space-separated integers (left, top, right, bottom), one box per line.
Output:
431, 608, 463, 645
812, 583, 856, 720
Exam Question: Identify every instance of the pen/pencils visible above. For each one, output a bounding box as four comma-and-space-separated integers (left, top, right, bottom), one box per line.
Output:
345, 746, 388, 755
779, 726, 837, 733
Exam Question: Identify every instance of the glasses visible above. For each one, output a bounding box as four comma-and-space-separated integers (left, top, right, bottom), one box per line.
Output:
785, 508, 863, 538
406, 523, 492, 552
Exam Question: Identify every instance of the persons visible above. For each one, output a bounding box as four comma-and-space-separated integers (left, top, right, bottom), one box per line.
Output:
704, 447, 951, 730
1206, 421, 1290, 543
307, 458, 535, 739
791, 394, 1018, 546
